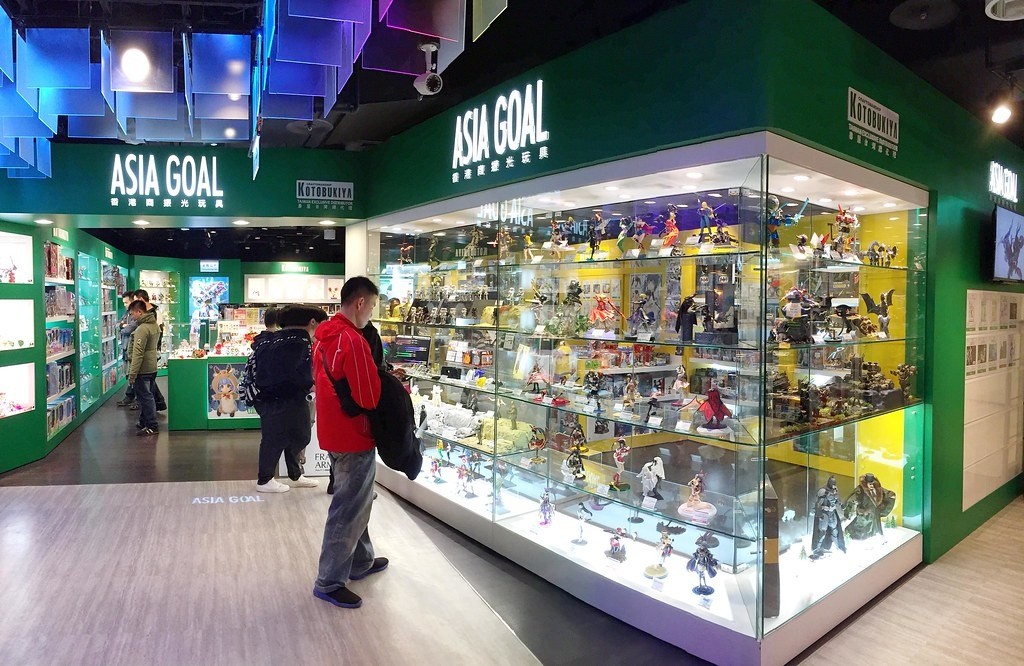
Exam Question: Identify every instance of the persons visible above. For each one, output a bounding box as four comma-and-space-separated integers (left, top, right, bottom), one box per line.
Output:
125, 300, 159, 435
116, 289, 167, 411
251, 304, 328, 490
755, 194, 798, 253
845, 473, 896, 541
812, 475, 847, 556
868, 241, 894, 267
890, 363, 917, 401
312, 275, 389, 608
782, 286, 818, 320
835, 202, 854, 252
400, 199, 734, 590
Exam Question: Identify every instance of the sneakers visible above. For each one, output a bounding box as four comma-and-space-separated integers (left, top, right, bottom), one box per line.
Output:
349, 556, 388, 580
127, 398, 140, 410
116, 397, 134, 406
156, 400, 167, 411
256, 476, 290, 493
313, 586, 363, 609
287, 475, 320, 488
135, 420, 146, 428
135, 423, 159, 437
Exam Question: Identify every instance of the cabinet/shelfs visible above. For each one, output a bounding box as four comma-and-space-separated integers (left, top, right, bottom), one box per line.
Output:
366, 126, 925, 666
42, 244, 182, 455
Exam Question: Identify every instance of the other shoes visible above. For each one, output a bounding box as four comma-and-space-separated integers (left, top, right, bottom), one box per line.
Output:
327, 482, 335, 494
372, 492, 377, 500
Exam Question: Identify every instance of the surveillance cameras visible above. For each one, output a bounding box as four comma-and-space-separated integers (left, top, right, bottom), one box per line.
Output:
413, 73, 443, 96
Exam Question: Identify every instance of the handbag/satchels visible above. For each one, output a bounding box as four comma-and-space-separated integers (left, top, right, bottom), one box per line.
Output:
234, 340, 269, 407
332, 377, 366, 417
